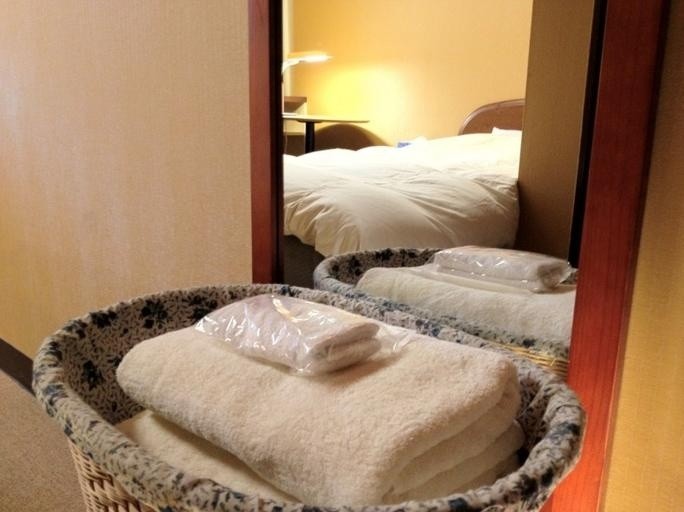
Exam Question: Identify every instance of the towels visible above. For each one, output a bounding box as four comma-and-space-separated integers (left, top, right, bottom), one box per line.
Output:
357, 267, 575, 350
112, 404, 524, 508
204, 294, 383, 372
116, 313, 524, 507
434, 244, 575, 292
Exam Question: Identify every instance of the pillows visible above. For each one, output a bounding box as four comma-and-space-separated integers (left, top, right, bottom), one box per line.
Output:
491, 127, 522, 175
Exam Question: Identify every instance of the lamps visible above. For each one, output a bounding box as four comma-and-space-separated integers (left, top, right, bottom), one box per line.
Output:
280, 49, 333, 79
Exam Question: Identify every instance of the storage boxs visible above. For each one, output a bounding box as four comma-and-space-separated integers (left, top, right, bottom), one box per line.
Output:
31, 279, 588, 512
313, 246, 568, 385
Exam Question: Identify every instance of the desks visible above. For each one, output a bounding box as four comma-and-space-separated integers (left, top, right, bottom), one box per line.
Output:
281, 112, 369, 154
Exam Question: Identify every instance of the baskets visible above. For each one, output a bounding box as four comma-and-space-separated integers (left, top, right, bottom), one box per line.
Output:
488, 339, 569, 382
66, 441, 151, 511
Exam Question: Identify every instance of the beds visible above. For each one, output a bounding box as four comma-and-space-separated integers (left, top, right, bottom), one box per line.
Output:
281, 99, 527, 255
